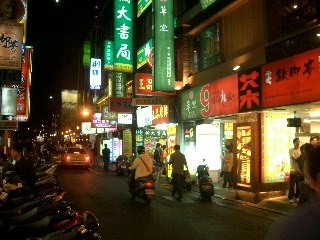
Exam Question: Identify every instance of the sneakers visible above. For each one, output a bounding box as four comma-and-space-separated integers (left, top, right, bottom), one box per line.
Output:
289, 199, 294, 202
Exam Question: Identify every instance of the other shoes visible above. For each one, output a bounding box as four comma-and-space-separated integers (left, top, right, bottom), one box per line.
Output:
180, 198, 183, 200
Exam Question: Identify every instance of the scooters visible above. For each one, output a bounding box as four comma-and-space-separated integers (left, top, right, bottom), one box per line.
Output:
0, 137, 101, 240
126, 164, 156, 206
116, 154, 130, 177
191, 158, 215, 203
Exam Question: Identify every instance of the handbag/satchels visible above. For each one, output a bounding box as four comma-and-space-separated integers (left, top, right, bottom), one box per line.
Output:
220, 171, 223, 177
150, 175, 155, 181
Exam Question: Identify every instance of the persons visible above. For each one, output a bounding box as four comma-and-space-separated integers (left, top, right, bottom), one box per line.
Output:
154, 143, 161, 179
0, 137, 98, 202
266, 137, 320, 240
221, 145, 233, 188
102, 144, 111, 173
161, 146, 167, 176
129, 146, 153, 194
167, 145, 189, 200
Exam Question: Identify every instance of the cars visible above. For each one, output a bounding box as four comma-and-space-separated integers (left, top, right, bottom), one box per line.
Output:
61, 147, 90, 172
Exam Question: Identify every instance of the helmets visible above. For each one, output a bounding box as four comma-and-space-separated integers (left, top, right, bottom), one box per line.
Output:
137, 146, 145, 154
5, 171, 19, 185
